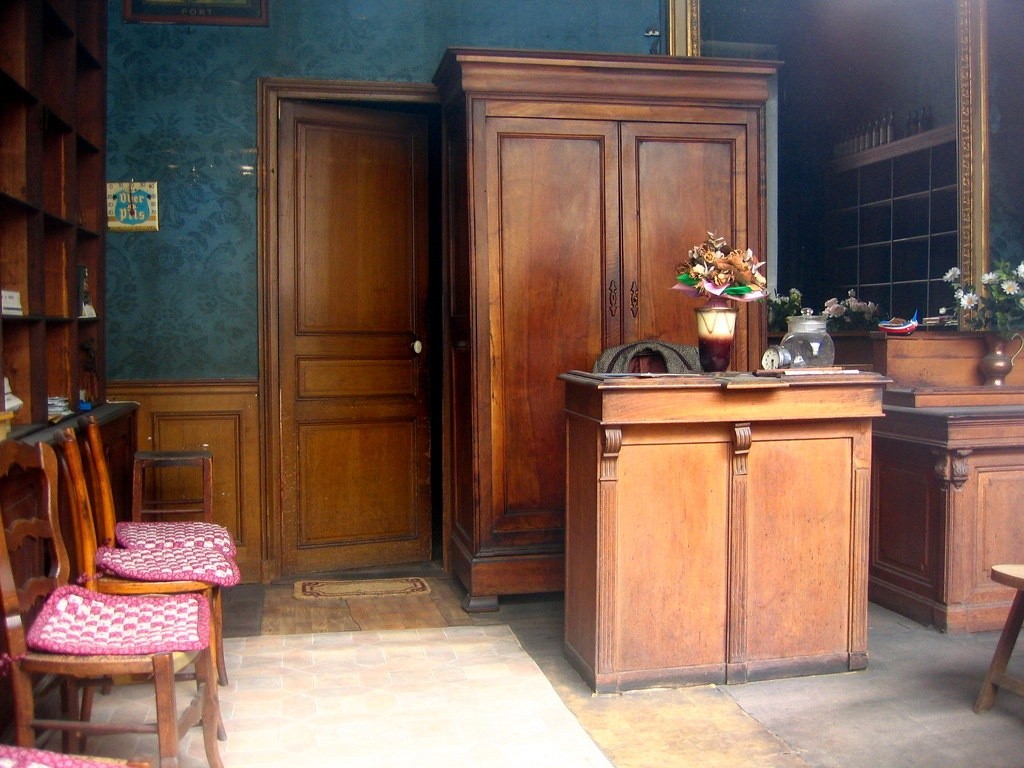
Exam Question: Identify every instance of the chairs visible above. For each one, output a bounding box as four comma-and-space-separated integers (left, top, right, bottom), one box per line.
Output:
0, 415, 241, 768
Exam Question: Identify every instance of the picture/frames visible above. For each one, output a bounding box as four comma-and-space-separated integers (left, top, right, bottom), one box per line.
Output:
122, 1, 269, 28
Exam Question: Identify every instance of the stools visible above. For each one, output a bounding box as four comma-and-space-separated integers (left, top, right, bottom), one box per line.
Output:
594, 338, 705, 374
973, 563, 1023, 713
129, 449, 213, 527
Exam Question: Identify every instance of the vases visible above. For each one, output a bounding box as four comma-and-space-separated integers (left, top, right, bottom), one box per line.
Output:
980, 330, 1024, 386
694, 298, 739, 371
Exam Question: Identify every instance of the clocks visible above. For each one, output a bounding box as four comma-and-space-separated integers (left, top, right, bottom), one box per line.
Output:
761, 344, 791, 371
106, 180, 159, 232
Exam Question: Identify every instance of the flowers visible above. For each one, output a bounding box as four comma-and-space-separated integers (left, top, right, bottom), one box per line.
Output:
938, 260, 1024, 341
822, 288, 883, 330
765, 288, 806, 331
670, 230, 771, 303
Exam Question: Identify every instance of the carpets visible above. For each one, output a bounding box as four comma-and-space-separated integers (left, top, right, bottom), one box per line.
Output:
37, 624, 616, 767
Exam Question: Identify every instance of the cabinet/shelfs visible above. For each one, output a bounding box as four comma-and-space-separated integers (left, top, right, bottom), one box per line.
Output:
0, 0, 140, 624
432, 48, 788, 612
557, 368, 894, 693
